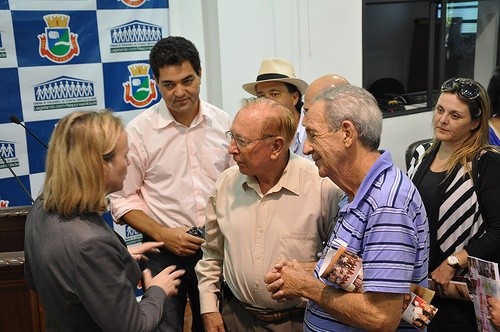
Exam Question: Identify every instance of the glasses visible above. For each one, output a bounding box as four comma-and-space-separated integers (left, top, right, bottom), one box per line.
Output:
225, 131, 274, 149
441, 78, 481, 100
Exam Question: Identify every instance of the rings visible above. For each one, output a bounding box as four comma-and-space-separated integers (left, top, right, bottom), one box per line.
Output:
486, 314, 491, 320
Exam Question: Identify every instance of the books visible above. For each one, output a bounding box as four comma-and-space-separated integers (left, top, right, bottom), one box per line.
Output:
320, 244, 438, 330
428, 256, 499, 302
471, 273, 500, 332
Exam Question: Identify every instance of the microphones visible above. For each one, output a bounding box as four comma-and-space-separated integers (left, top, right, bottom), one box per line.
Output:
10, 116, 47, 148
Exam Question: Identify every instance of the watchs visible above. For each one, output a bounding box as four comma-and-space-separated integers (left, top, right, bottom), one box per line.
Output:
447, 255, 463, 271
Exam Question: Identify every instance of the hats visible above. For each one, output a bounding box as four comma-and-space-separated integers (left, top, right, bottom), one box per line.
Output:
242, 59, 308, 96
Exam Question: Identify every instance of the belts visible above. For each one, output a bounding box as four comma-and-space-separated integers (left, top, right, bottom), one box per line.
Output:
222, 281, 305, 323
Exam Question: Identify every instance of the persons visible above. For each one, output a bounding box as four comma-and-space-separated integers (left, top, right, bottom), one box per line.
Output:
470, 258, 478, 271
242, 58, 315, 163
404, 293, 412, 301
109, 35, 238, 332
265, 65, 500, 332
370, 78, 405, 107
194, 96, 346, 332
340, 256, 355, 271
24, 109, 184, 332
412, 318, 427, 330
